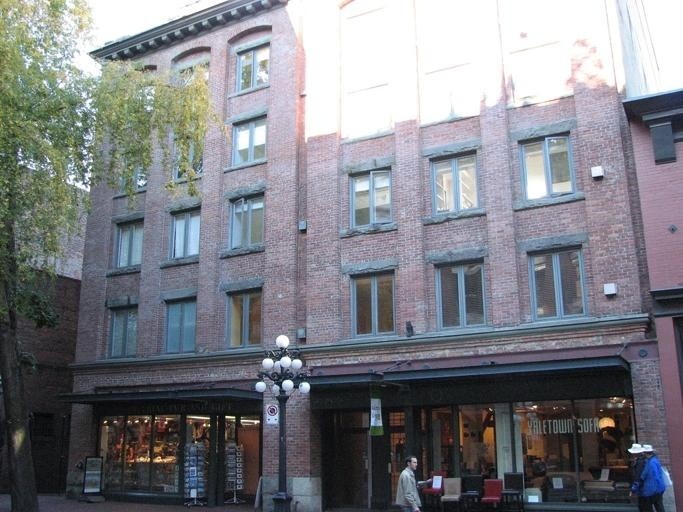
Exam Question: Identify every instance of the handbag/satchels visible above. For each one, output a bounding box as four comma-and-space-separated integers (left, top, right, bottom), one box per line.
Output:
660, 467, 673, 489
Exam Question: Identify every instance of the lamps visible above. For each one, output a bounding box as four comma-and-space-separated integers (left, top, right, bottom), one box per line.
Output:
598, 416, 615, 432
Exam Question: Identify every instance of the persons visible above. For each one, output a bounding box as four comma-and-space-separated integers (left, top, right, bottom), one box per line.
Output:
641, 443, 666, 512
395, 454, 422, 512
530, 457, 548, 489
627, 442, 660, 512
394, 438, 405, 463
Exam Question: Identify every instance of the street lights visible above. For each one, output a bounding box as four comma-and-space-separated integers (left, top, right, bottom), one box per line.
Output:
255, 335, 311, 512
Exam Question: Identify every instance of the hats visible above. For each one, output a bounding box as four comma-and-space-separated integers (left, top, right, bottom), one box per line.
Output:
642, 444, 654, 453
627, 443, 644, 454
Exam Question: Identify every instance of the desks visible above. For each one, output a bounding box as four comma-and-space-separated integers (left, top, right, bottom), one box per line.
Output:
583, 479, 614, 502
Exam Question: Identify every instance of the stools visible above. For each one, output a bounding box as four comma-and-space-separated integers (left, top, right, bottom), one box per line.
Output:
615, 482, 633, 504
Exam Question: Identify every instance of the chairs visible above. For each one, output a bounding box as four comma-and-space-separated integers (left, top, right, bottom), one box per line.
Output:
422, 471, 524, 512
